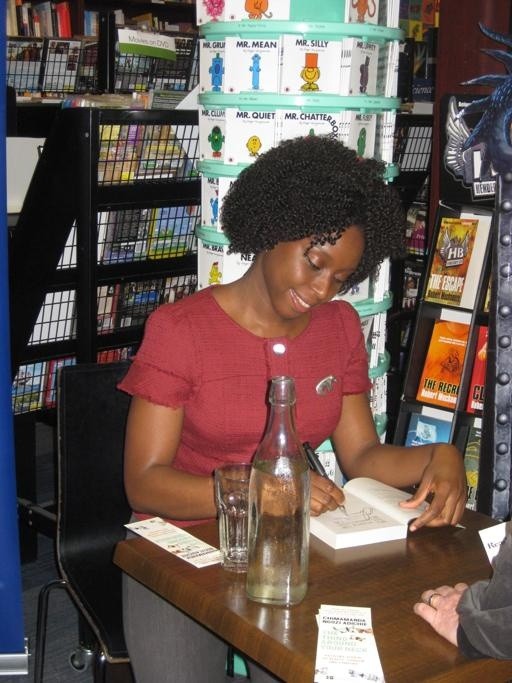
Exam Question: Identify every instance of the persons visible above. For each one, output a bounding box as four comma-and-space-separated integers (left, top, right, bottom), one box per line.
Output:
416, 519, 512, 663
116, 138, 469, 683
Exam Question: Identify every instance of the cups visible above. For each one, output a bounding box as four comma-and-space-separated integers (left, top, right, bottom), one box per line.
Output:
215, 462, 269, 573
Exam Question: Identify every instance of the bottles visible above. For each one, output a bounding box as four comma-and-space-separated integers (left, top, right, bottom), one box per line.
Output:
243, 375, 312, 608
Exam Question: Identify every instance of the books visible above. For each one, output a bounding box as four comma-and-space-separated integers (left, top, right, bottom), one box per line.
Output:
415, 320, 490, 413
6, 0, 99, 93
403, 411, 455, 450
308, 478, 465, 550
423, 212, 492, 309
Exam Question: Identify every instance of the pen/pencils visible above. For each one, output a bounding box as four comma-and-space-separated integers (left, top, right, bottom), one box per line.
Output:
301, 441, 347, 518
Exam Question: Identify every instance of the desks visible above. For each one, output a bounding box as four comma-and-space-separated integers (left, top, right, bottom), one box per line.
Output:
113, 484, 512, 683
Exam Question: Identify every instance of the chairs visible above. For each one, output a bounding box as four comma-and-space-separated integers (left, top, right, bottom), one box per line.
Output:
31, 355, 131, 661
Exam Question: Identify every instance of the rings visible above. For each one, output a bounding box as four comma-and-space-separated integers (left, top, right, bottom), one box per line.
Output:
428, 593, 441, 607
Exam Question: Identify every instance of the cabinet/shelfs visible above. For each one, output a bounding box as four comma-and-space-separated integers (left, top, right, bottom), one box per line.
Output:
198, 1, 407, 486
2, 2, 436, 417
392, 174, 512, 522
